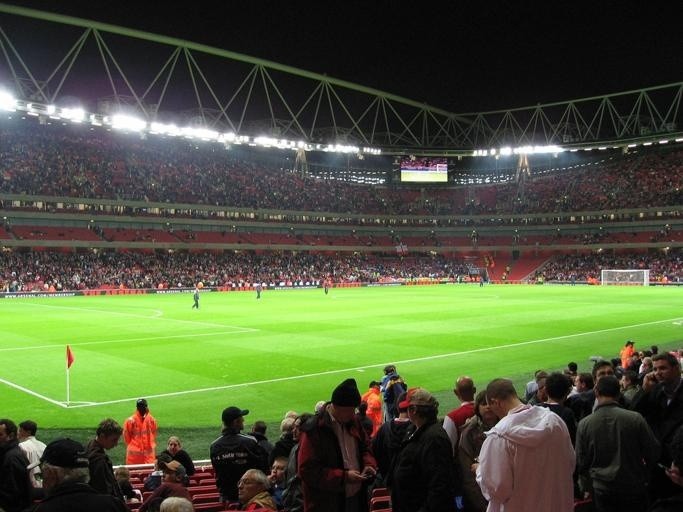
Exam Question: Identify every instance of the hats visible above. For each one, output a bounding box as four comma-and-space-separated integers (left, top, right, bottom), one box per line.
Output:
26, 437, 89, 471
156, 458, 186, 476
398, 386, 439, 411
627, 340, 636, 345
221, 406, 249, 422
136, 399, 148, 409
331, 377, 364, 405
368, 380, 382, 387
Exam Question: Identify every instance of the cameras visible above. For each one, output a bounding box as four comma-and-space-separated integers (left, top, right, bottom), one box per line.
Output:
357, 471, 372, 478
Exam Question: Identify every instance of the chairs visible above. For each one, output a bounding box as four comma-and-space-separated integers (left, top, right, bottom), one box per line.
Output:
2, 249, 683, 295
402, 144, 681, 242
369, 488, 392, 512
573, 498, 593, 511
1, 115, 400, 247
125, 460, 239, 511
